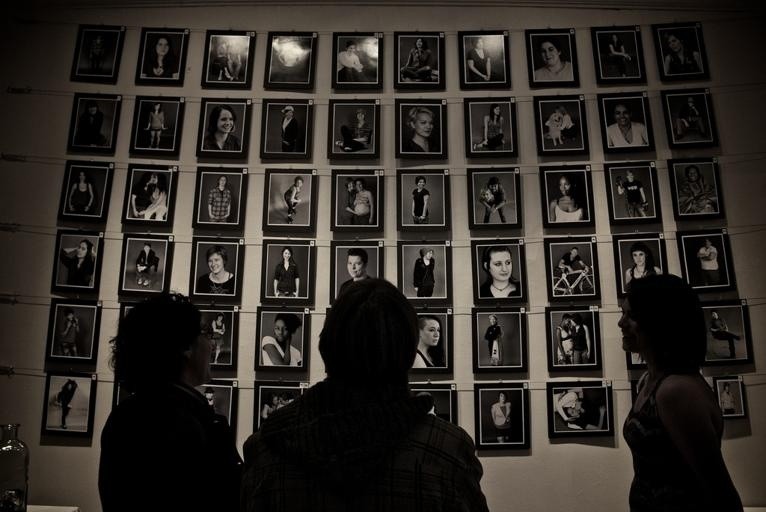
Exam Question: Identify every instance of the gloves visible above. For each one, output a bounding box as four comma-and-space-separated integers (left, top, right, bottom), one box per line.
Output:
644, 201, 649, 211
616, 176, 624, 186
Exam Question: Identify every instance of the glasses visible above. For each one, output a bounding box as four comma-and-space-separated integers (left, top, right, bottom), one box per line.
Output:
200, 323, 213, 339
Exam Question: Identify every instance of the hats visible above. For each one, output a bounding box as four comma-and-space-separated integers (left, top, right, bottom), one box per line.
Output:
205, 387, 215, 393
486, 177, 501, 185
281, 105, 295, 114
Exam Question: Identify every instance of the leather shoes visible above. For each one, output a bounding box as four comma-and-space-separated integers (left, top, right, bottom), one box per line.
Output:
62, 425, 67, 429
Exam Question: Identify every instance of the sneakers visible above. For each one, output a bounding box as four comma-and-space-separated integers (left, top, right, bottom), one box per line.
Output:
335, 141, 343, 147
136, 279, 151, 286
288, 218, 296, 224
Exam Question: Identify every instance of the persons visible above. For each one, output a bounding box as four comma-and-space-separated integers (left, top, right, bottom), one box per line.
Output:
664, 31, 744, 417
607, 35, 663, 280
97, 293, 242, 512
262, 106, 301, 416
466, 38, 517, 441
244, 277, 491, 511
535, 42, 606, 429
131, 35, 180, 286
57, 32, 108, 429
198, 39, 241, 412
335, 41, 373, 289
401, 36, 446, 416
615, 275, 746, 511
